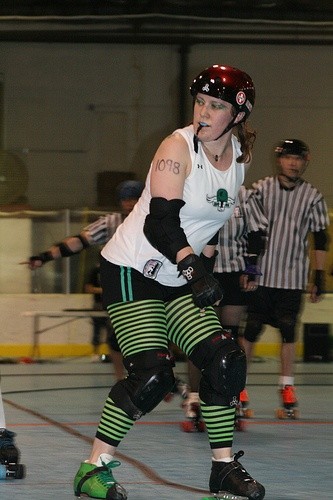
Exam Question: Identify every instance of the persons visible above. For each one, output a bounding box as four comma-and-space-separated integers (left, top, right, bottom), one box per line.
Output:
74, 64, 265, 500
182, 187, 269, 433
85, 259, 112, 361
238, 139, 329, 419
19, 181, 187, 402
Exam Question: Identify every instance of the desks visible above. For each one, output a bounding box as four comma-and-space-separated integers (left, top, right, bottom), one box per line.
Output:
20, 309, 110, 364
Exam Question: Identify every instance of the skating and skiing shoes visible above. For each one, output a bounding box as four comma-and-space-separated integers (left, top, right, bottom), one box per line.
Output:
206, 450, 266, 500
276, 385, 300, 418
234, 386, 253, 418
73, 454, 128, 500
234, 416, 246, 432
179, 392, 206, 432
0, 427, 24, 480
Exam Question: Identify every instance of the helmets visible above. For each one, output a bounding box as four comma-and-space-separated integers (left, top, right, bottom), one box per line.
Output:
273, 138, 311, 162
189, 64, 255, 120
118, 180, 143, 199
